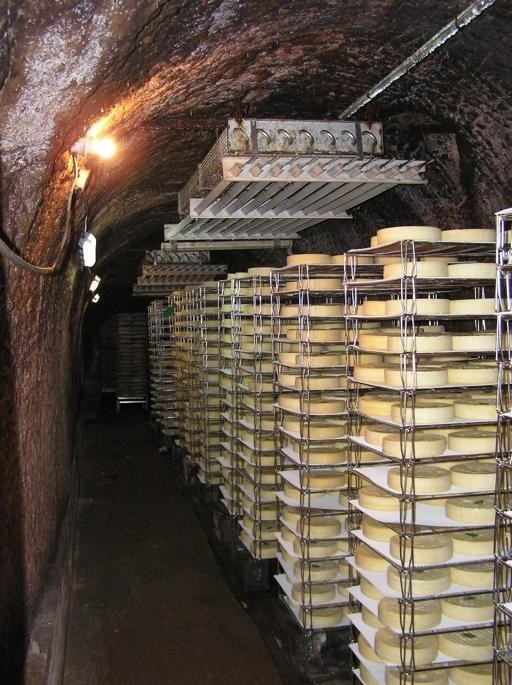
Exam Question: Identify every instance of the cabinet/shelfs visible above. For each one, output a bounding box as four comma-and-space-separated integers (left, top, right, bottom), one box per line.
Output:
109, 209, 511, 685
